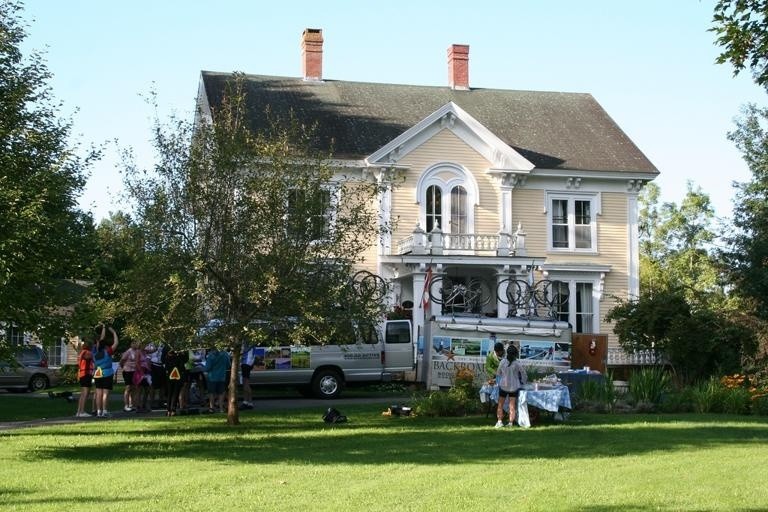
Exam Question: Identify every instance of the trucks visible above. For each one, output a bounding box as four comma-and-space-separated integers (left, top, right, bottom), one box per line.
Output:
142, 313, 415, 400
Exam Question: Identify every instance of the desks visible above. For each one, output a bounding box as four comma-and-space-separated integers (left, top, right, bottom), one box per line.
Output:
528, 371, 604, 394
486, 382, 567, 428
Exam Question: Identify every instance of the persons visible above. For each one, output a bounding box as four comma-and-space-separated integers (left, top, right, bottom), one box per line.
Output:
76, 321, 255, 418
484, 342, 506, 379
494, 345, 528, 428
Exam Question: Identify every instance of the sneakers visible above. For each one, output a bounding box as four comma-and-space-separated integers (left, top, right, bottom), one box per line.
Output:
494, 420, 514, 428
75, 399, 256, 419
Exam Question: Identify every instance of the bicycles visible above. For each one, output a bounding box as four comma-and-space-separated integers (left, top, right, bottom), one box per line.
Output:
428, 275, 570, 321
351, 270, 386, 309
